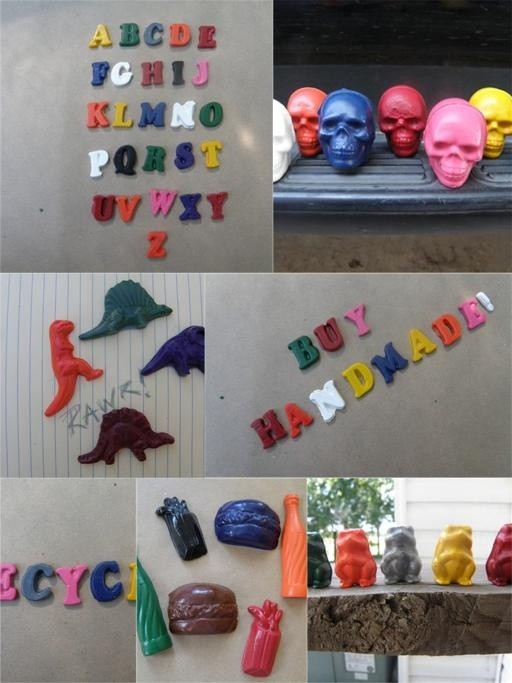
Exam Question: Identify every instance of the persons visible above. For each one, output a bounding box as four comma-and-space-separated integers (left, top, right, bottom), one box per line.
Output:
432, 525, 475, 584
486, 524, 512, 585
79, 280, 173, 339
381, 525, 421, 583
273, 84, 512, 189
308, 532, 331, 588
77, 408, 175, 465
334, 528, 376, 588
141, 326, 204, 375
45, 320, 103, 417
